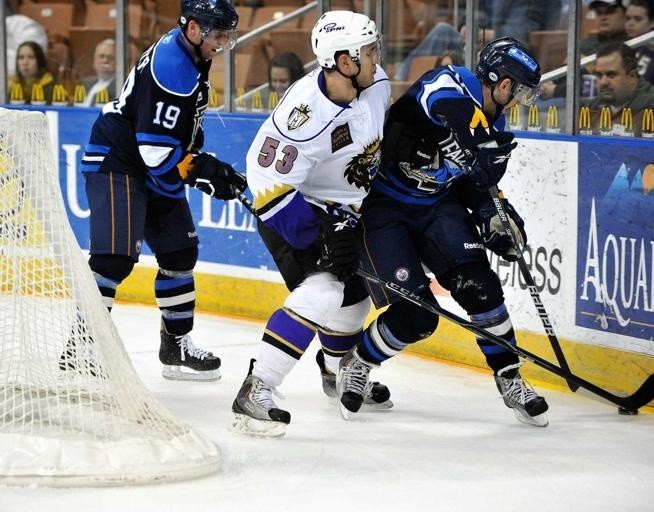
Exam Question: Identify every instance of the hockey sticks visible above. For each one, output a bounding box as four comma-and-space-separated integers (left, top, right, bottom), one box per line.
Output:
357, 269, 654, 409
492, 189, 628, 406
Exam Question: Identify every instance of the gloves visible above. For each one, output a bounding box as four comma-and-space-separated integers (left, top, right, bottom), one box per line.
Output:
179, 153, 248, 198
479, 197, 529, 262
472, 143, 518, 192
331, 222, 359, 279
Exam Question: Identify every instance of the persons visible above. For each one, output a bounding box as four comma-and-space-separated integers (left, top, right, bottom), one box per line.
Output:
398, 0, 652, 131
329, 31, 551, 418
5, 1, 117, 104
54, 0, 249, 376
268, 52, 308, 98
227, 8, 404, 422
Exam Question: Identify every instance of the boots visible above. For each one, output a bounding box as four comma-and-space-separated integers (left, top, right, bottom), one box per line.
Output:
231, 359, 291, 425
339, 346, 380, 413
314, 349, 390, 404
492, 370, 549, 417
61, 322, 113, 378
156, 317, 222, 371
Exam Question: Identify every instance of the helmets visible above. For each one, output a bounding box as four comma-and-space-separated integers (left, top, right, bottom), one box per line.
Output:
475, 35, 542, 108
174, 0, 239, 50
311, 8, 382, 69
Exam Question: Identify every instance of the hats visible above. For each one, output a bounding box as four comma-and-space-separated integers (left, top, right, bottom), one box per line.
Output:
588, 0, 632, 13
